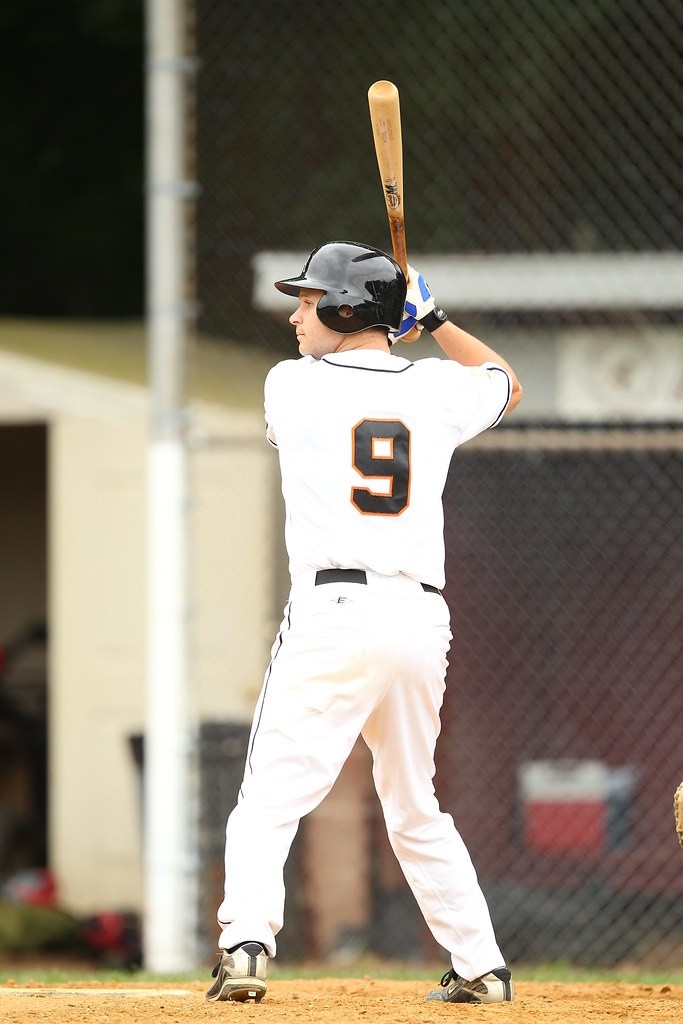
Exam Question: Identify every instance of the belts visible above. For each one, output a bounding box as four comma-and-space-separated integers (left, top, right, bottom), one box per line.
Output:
314, 568, 442, 595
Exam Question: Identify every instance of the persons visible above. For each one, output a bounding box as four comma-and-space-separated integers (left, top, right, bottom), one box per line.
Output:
208, 240, 527, 1003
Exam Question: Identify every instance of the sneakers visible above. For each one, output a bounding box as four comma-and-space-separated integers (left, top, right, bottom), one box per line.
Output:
426, 968, 513, 1004
205, 941, 269, 1002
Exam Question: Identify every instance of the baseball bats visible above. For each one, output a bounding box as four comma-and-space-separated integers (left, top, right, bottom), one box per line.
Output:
367, 80, 421, 343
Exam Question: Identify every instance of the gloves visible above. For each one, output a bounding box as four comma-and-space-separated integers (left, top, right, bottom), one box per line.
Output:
405, 264, 448, 334
387, 313, 418, 348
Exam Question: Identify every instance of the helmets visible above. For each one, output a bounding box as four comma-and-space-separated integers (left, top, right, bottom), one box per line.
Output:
275, 240, 407, 334
